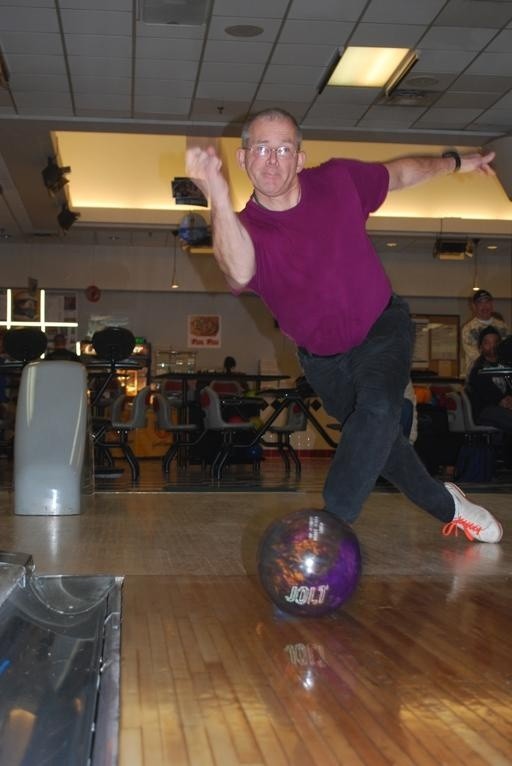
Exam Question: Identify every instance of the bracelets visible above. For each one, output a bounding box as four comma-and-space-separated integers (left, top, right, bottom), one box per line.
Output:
441, 150, 463, 177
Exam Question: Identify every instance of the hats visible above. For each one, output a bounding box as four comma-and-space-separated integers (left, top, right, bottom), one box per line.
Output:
472, 289, 491, 303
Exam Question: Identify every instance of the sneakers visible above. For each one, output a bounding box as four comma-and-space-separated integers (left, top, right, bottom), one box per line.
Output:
443, 482, 503, 544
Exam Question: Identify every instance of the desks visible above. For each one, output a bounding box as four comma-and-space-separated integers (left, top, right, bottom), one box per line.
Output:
154, 373, 291, 483
480, 368, 511, 407
0, 359, 143, 480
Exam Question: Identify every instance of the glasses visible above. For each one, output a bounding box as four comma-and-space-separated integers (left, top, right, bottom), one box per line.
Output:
243, 145, 297, 158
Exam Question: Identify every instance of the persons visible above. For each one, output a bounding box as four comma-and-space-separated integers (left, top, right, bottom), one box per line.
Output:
464, 326, 511, 470
182, 105, 507, 545
459, 288, 511, 387
